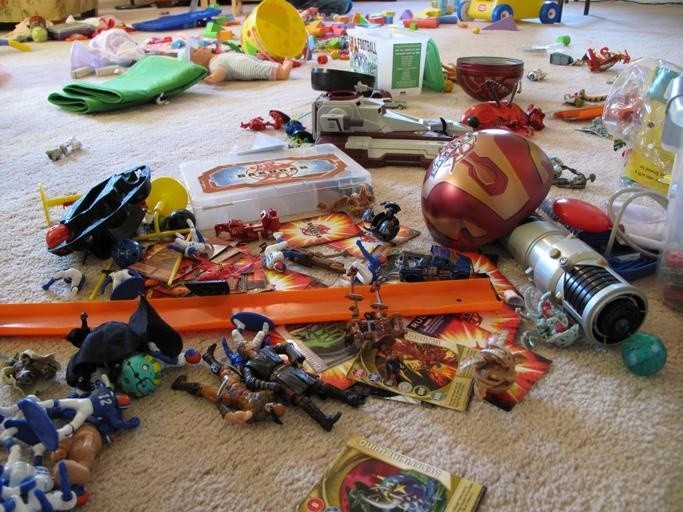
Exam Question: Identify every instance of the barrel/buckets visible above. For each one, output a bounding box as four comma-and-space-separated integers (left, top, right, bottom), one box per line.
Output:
240, 0, 309, 67
